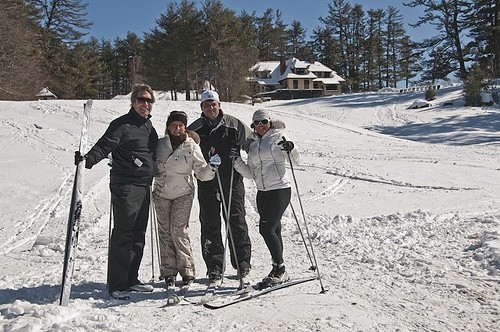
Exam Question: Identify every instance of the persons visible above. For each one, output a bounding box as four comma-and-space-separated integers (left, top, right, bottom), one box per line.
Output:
151, 110, 222, 288
185, 91, 254, 281
228, 110, 301, 286
75, 83, 159, 299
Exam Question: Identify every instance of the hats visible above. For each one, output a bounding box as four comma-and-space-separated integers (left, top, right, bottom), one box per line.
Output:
253, 109, 272, 122
200, 90, 220, 111
166, 110, 187, 129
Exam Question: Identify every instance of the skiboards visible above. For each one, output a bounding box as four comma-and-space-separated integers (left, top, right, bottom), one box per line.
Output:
163, 273, 190, 303
59, 96, 94, 307
198, 275, 317, 311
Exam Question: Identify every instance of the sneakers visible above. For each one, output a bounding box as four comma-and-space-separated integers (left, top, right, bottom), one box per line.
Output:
110, 290, 131, 300
207, 266, 222, 281
165, 276, 176, 286
263, 265, 289, 283
126, 283, 153, 292
237, 266, 252, 279
182, 277, 194, 285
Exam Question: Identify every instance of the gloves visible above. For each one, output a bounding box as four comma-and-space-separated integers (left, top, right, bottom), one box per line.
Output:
209, 150, 221, 172
277, 141, 294, 151
229, 148, 238, 160
74, 151, 87, 168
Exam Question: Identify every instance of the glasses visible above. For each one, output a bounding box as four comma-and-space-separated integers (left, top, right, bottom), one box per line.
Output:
135, 96, 154, 103
254, 118, 270, 126
